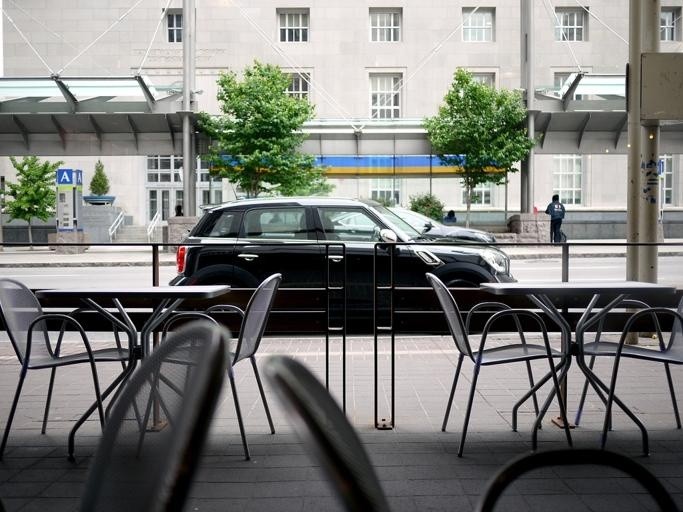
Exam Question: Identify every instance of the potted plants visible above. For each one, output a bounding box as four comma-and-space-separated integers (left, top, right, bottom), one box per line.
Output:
83, 159, 116, 204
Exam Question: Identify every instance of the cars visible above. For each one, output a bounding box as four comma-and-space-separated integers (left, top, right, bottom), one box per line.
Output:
326, 206, 498, 248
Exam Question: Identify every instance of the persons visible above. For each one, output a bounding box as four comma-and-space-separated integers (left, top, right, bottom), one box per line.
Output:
546, 193, 565, 244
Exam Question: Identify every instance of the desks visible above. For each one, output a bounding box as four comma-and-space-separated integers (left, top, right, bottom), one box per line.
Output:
479, 281, 673, 457
29, 281, 232, 461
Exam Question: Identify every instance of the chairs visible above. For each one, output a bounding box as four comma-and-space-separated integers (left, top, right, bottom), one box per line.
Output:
135, 268, 283, 459
568, 287, 682, 455
74, 312, 232, 512
262, 352, 678, 511
423, 272, 572, 460
0, 278, 140, 456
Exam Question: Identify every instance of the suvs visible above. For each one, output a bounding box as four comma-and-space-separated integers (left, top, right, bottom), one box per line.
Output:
166, 196, 518, 338
